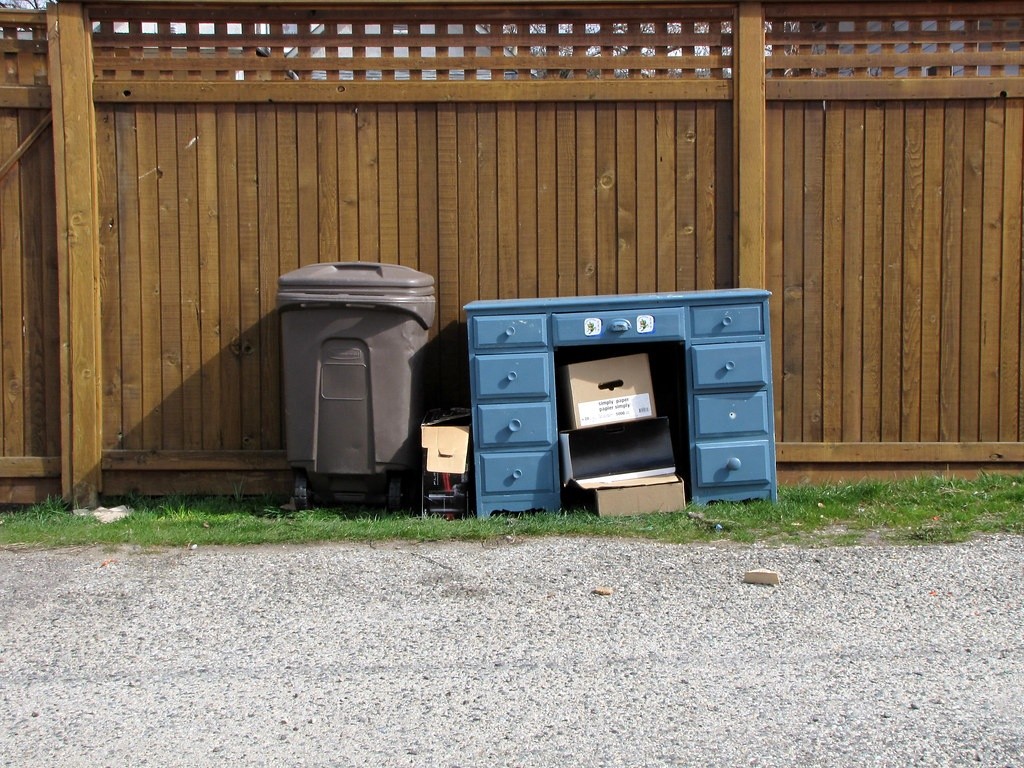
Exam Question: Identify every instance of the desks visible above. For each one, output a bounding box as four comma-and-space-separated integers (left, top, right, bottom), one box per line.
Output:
459, 288, 779, 520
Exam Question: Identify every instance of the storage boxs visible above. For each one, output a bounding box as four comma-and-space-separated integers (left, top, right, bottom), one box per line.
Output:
420, 406, 474, 518
576, 472, 685, 518
558, 353, 657, 429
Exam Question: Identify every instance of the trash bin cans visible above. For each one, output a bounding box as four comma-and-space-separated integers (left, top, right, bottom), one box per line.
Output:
273, 260, 437, 514
